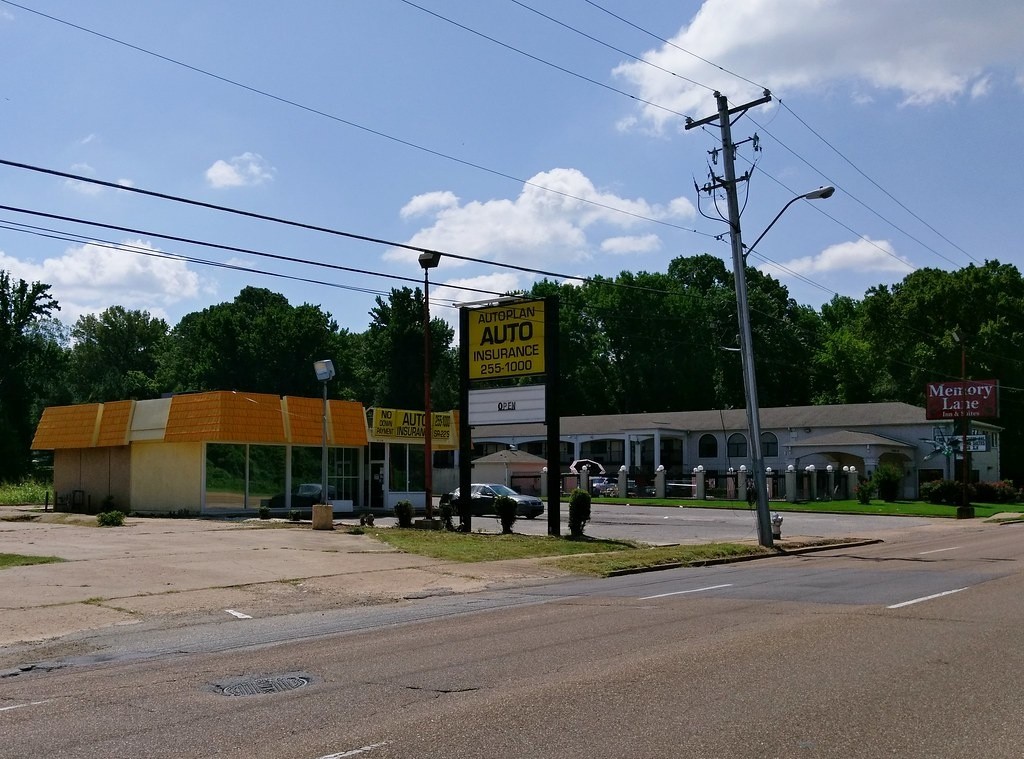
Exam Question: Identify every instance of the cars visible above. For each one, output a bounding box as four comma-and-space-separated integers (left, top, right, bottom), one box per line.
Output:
270, 483, 343, 509
448, 483, 545, 519
591, 477, 619, 496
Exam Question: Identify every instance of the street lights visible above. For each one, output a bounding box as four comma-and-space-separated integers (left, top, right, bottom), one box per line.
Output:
731, 186, 837, 549
311, 359, 336, 531
414, 250, 446, 530
